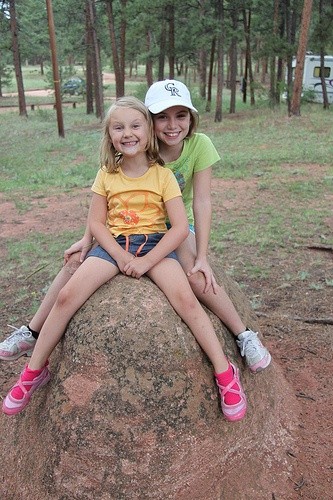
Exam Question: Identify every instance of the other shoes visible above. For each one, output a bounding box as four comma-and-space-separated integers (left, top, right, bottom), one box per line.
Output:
215, 360, 247, 422
2, 366, 52, 414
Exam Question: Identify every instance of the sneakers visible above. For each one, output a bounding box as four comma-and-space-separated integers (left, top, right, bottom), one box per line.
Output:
236, 328, 272, 374
0, 325, 37, 360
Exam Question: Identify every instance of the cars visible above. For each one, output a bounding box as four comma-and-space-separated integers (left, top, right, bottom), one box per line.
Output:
61, 76, 87, 97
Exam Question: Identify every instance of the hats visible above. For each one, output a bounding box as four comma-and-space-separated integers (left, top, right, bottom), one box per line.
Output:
143, 79, 199, 115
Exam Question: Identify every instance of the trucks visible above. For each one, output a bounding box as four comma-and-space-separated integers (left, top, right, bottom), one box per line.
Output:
282, 51, 333, 106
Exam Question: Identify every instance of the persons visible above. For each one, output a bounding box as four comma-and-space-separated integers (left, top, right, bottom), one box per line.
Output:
1, 79, 273, 374
1, 96, 249, 423
